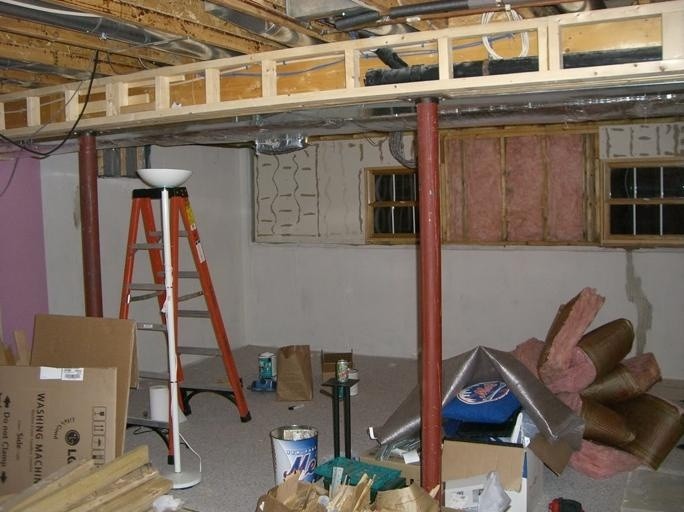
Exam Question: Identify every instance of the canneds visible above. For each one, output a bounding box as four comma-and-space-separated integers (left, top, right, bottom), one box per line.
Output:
336, 359, 349, 382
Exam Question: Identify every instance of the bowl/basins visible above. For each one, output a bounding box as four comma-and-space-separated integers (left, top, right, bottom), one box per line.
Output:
137, 168, 192, 188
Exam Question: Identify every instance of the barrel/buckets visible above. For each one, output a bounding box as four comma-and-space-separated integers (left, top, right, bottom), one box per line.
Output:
268, 423, 319, 483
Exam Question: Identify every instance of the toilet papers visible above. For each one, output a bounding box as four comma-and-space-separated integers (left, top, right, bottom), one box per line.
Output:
147, 383, 190, 426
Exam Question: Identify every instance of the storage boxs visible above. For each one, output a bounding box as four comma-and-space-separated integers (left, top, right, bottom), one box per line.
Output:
276, 342, 315, 403
440, 429, 572, 512
320, 348, 353, 381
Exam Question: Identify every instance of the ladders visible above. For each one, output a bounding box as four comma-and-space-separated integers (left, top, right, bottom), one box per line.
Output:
119, 186, 252, 465
